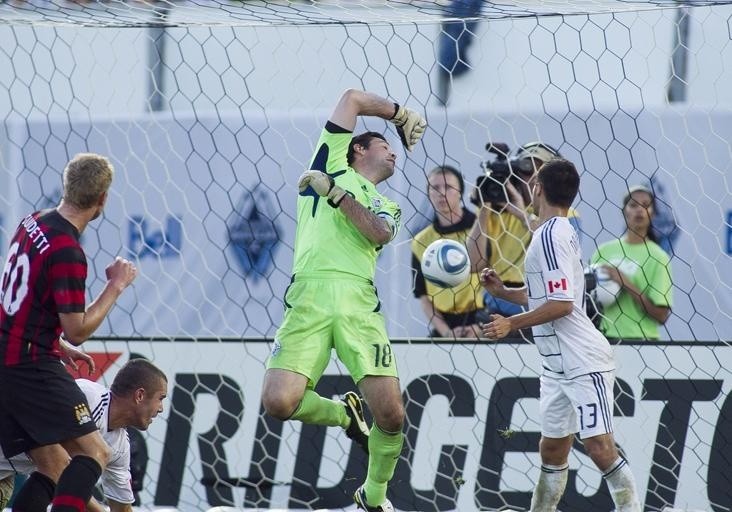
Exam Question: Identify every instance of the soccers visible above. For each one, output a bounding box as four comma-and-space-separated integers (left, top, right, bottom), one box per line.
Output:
421, 238, 471, 289
584, 263, 622, 306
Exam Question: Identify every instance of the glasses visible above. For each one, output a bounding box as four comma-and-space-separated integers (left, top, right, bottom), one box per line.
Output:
518, 142, 557, 155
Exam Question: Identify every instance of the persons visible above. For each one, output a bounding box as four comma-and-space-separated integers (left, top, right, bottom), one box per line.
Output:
408, 164, 489, 338
262, 86, 429, 511
462, 142, 586, 335
2, 357, 168, 510
585, 184, 676, 342
2, 151, 141, 511
477, 158, 642, 511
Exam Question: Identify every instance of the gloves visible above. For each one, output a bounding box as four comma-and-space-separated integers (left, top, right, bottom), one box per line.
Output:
388, 103, 427, 153
297, 169, 347, 209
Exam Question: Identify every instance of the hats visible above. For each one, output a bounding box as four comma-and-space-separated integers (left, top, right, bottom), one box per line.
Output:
517, 141, 559, 163
623, 185, 654, 202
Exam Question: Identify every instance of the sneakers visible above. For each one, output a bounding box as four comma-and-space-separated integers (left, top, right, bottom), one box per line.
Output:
353, 486, 394, 512
340, 391, 370, 455
